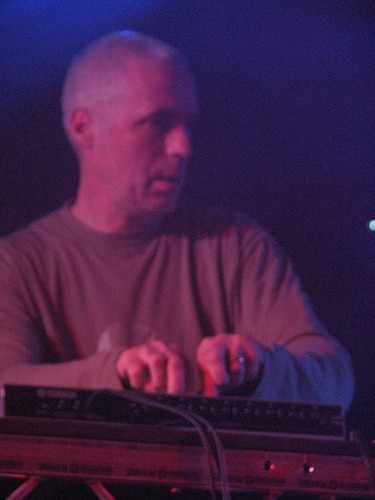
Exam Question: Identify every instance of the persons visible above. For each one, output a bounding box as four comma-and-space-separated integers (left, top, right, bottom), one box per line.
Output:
0, 28, 356, 416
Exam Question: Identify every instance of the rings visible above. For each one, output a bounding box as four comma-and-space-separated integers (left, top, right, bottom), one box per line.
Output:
230, 355, 244, 364
